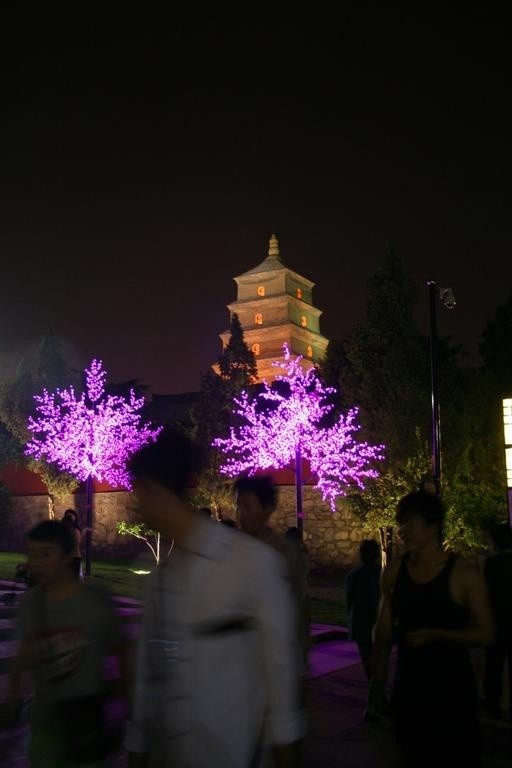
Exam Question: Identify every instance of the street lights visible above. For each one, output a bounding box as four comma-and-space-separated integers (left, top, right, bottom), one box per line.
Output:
426, 276, 459, 499
501, 397, 512, 531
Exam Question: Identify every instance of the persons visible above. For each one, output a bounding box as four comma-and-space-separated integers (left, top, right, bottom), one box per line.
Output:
109, 440, 309, 768
480, 524, 512, 716
234, 474, 307, 705
62, 509, 94, 585
11, 519, 138, 767
345, 538, 390, 718
371, 488, 496, 768
286, 523, 308, 580
